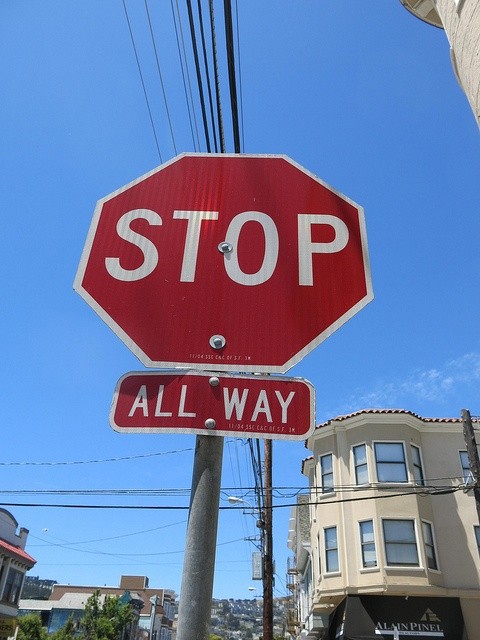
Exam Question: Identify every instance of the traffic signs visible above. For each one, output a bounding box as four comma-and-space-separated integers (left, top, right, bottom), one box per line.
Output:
109, 372, 316, 440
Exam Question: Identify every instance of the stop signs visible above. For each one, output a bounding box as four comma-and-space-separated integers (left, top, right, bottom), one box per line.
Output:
72, 151, 374, 374
